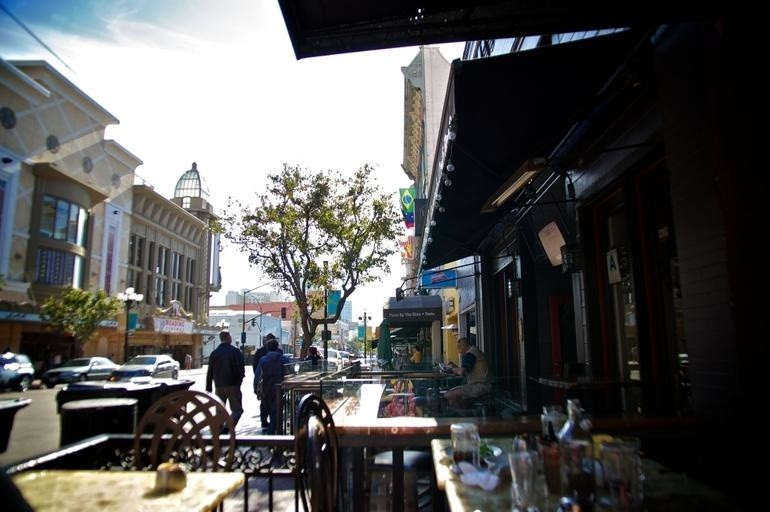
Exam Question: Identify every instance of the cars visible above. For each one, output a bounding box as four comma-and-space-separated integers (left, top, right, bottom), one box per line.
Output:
108, 354, 182, 384
0, 349, 36, 394
41, 354, 121, 390
317, 348, 375, 372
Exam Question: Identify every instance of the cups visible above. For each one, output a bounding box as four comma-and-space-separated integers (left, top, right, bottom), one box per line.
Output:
450, 398, 650, 512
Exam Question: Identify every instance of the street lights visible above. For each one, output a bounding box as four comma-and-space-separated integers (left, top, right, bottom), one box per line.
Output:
358, 312, 372, 363
113, 286, 145, 361
312, 260, 341, 371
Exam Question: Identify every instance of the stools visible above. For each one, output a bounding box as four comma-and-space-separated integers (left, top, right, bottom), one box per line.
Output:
470, 399, 491, 422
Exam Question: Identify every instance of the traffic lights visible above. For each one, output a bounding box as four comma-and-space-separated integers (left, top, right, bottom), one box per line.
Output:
253, 319, 256, 326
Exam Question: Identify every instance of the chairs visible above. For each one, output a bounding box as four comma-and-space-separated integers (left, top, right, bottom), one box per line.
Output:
294, 393, 340, 512
134, 390, 235, 512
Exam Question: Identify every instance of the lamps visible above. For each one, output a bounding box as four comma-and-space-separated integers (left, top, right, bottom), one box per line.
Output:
507, 277, 520, 299
560, 244, 586, 274
416, 115, 458, 274
479, 155, 545, 214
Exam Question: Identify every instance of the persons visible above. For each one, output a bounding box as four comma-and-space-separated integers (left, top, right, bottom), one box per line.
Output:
301, 346, 323, 372
409, 345, 422, 363
206, 331, 245, 435
184, 353, 192, 369
445, 337, 491, 417
253, 339, 296, 435
252, 333, 283, 429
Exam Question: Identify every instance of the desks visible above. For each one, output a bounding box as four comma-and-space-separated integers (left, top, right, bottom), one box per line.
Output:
404, 372, 457, 417
11, 468, 246, 511
0, 398, 33, 454
